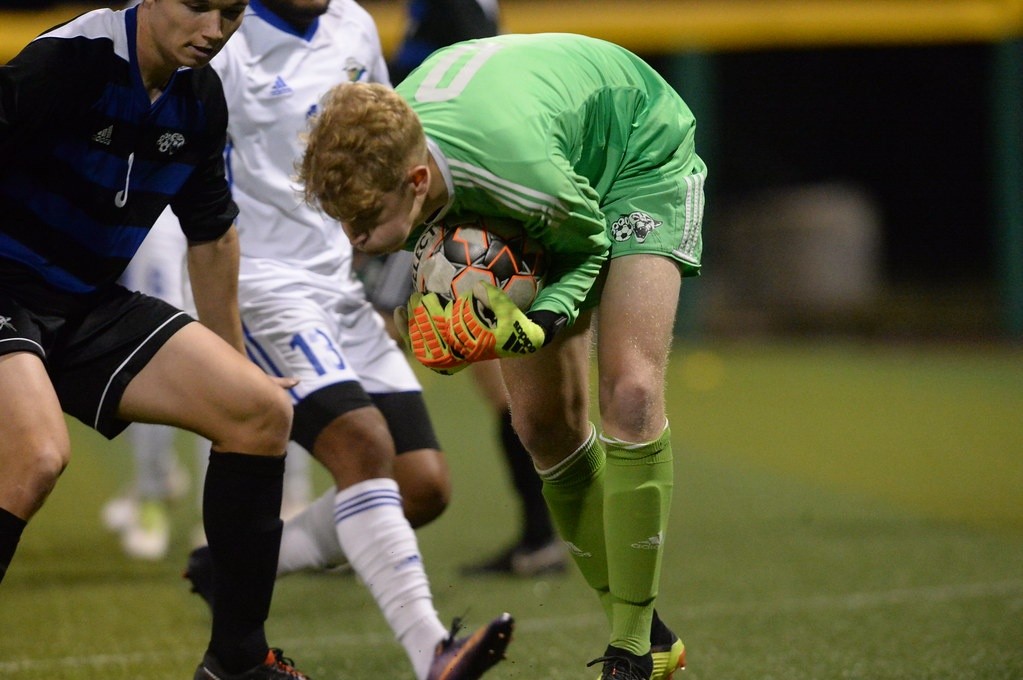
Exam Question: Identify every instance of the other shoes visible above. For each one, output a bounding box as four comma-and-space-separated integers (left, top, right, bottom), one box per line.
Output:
100, 497, 172, 562
479, 544, 569, 579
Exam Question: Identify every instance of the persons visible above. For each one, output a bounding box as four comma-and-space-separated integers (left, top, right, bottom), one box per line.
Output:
0, 1, 708, 680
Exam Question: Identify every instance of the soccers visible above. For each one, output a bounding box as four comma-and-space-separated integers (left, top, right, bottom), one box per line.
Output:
410, 224, 540, 314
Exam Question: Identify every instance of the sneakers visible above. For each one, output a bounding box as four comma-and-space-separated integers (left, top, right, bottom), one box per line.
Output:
650, 630, 686, 680
193, 647, 312, 680
587, 649, 654, 680
185, 548, 216, 615
424, 612, 517, 680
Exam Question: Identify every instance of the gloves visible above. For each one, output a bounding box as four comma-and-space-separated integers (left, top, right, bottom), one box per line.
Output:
392, 293, 472, 375
444, 279, 547, 362
